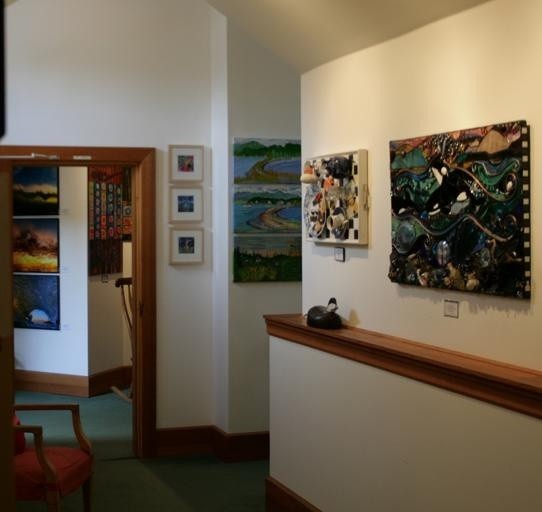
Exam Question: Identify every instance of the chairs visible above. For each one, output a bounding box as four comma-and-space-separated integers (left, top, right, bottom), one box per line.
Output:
12, 402, 93, 512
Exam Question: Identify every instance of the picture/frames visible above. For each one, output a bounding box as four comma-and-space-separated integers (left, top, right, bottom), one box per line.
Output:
168, 144, 204, 184
168, 226, 204, 265
168, 184, 203, 224
12, 165, 60, 330
